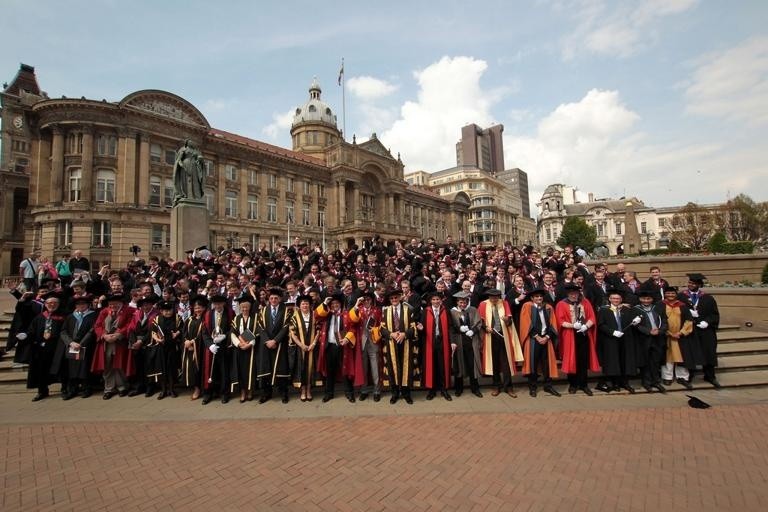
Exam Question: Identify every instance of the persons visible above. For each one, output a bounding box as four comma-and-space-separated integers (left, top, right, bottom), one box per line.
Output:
177, 138, 204, 199
9, 236, 721, 405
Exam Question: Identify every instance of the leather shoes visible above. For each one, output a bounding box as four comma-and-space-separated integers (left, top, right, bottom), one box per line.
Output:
565, 377, 720, 396
323, 395, 334, 402
473, 391, 482, 397
441, 390, 451, 401
31, 392, 49, 401
404, 396, 413, 403
374, 394, 380, 402
359, 393, 368, 401
59, 382, 313, 405
455, 389, 463, 396
544, 388, 558, 396
347, 396, 355, 403
390, 396, 399, 404
530, 390, 536, 396
426, 392, 436, 400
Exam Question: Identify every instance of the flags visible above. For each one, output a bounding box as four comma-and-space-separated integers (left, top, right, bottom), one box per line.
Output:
338, 61, 343, 86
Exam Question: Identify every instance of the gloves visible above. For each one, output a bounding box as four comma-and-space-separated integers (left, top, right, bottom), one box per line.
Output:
697, 320, 708, 329
209, 344, 219, 354
460, 325, 474, 337
689, 309, 699, 318
613, 330, 624, 337
632, 316, 641, 326
574, 321, 588, 333
15, 333, 27, 340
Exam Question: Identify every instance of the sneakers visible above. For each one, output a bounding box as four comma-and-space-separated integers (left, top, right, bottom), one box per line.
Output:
507, 391, 517, 398
491, 389, 499, 396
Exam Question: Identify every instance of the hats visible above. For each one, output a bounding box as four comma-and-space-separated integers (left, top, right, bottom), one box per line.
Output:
686, 394, 710, 409
40, 273, 707, 305
